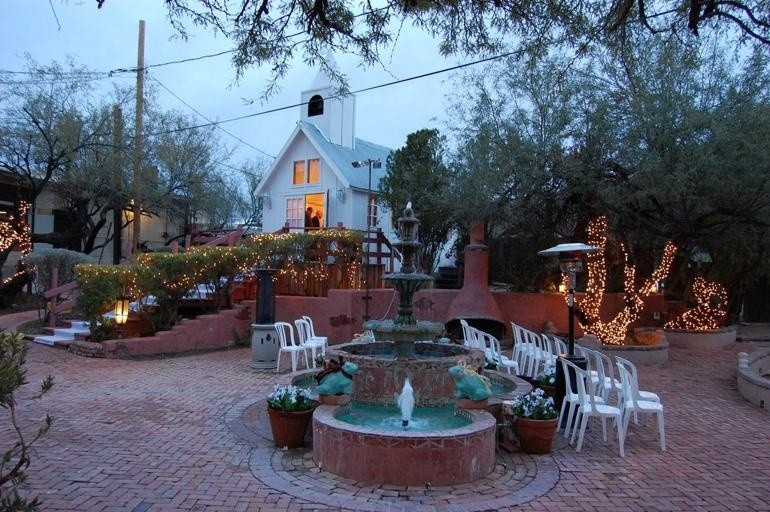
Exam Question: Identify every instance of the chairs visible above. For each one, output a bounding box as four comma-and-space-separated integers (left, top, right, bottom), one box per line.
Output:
273, 315, 328, 374
460, 316, 667, 458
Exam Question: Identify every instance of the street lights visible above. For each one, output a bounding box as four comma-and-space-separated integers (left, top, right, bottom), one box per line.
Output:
350, 155, 383, 328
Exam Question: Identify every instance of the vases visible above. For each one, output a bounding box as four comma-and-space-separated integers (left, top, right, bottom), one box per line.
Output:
267, 401, 318, 448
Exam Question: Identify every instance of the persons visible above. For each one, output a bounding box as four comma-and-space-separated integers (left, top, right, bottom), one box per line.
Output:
312, 209, 323, 227
306, 207, 314, 227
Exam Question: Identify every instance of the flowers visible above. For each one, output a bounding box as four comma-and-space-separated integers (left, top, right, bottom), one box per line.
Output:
266, 381, 314, 410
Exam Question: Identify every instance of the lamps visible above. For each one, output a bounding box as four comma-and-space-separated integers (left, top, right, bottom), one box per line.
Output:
115, 294, 130, 326
